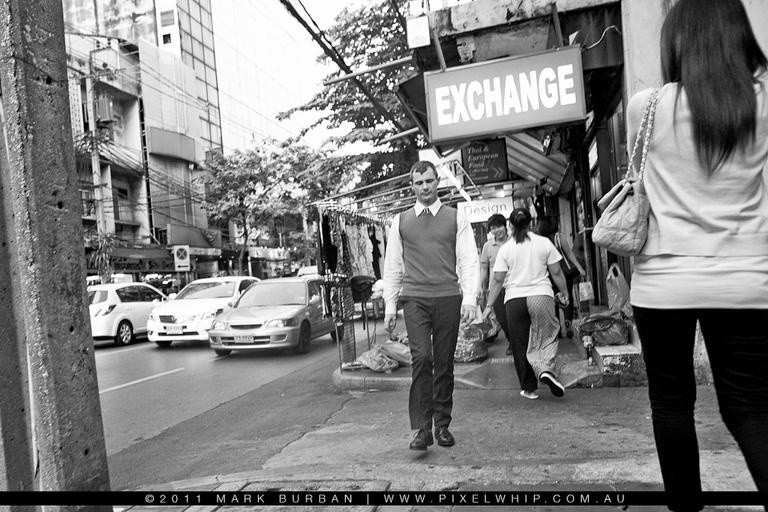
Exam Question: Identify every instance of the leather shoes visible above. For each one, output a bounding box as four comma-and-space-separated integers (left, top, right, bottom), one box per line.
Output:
435, 426, 454, 445
410, 430, 433, 450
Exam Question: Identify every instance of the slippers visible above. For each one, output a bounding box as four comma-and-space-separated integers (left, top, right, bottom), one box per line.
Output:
540, 372, 564, 397
520, 390, 539, 399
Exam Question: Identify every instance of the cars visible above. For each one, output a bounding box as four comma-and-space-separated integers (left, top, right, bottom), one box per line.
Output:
88, 283, 176, 346
146, 276, 262, 347
209, 276, 348, 355
349, 296, 402, 320
295, 265, 337, 277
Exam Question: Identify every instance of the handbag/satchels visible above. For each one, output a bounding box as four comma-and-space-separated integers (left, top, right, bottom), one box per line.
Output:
591, 178, 649, 257
556, 246, 579, 278
579, 276, 594, 302
454, 329, 488, 362
580, 318, 628, 346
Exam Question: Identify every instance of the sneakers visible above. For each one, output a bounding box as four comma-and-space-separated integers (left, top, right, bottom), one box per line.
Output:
506, 343, 512, 355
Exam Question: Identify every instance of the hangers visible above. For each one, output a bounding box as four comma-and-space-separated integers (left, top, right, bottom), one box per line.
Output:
318, 273, 353, 290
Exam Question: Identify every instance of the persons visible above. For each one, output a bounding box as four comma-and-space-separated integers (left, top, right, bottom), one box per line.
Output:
384, 162, 480, 451
482, 213, 514, 355
484, 208, 567, 397
538, 216, 586, 338
593, 1, 768, 512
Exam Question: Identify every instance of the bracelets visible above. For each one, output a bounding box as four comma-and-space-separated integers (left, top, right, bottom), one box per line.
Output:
485, 304, 493, 307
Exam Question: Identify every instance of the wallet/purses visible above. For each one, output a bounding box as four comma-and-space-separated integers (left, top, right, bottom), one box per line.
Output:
556, 292, 566, 305
